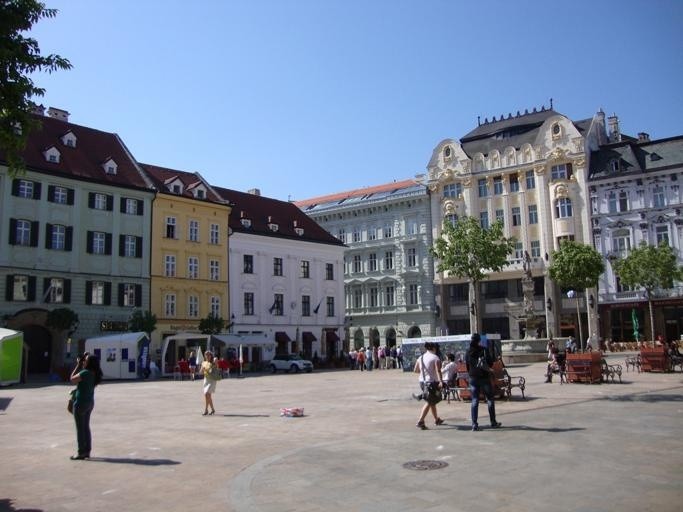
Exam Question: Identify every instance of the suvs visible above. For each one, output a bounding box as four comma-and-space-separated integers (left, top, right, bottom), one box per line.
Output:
269, 353, 314, 373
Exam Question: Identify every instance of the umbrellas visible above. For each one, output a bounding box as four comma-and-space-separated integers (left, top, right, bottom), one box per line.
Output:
631, 307, 642, 338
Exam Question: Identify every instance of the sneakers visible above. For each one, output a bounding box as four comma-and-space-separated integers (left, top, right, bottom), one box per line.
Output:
417, 421, 429, 430
491, 422, 502, 428
435, 417, 445, 424
472, 424, 482, 431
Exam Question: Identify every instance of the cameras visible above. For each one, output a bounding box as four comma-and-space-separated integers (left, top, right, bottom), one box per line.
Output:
77, 352, 88, 369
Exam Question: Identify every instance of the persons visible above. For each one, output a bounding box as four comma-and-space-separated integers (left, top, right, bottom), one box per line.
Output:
411, 333, 502, 431
544, 336, 606, 383
199, 350, 217, 415
188, 351, 197, 381
342, 344, 403, 371
69, 350, 103, 459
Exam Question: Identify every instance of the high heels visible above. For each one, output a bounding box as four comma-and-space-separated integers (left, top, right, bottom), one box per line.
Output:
70, 454, 84, 460
202, 411, 209, 415
85, 453, 91, 459
209, 411, 215, 415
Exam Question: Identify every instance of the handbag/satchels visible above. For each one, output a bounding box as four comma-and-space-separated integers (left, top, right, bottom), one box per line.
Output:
68, 397, 74, 414
423, 382, 442, 405
209, 364, 222, 379
475, 348, 495, 380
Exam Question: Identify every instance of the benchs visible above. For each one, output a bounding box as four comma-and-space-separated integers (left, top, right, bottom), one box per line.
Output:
443, 346, 683, 403
